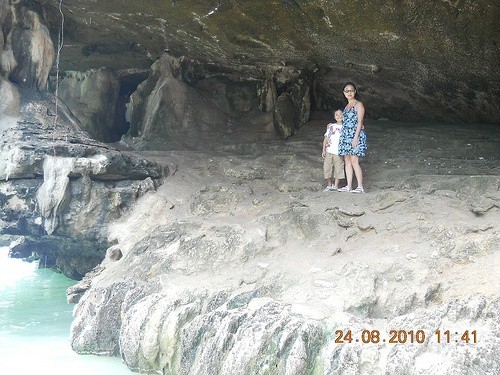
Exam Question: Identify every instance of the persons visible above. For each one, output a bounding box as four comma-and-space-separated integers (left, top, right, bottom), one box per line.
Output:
321, 109, 364, 193
338, 84, 367, 193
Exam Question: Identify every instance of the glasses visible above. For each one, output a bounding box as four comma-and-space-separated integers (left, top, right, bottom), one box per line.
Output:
344, 89, 355, 92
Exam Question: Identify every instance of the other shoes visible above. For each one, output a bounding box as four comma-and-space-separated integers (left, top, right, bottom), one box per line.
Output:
352, 187, 365, 193
324, 186, 330, 191
331, 184, 338, 190
339, 186, 352, 191
